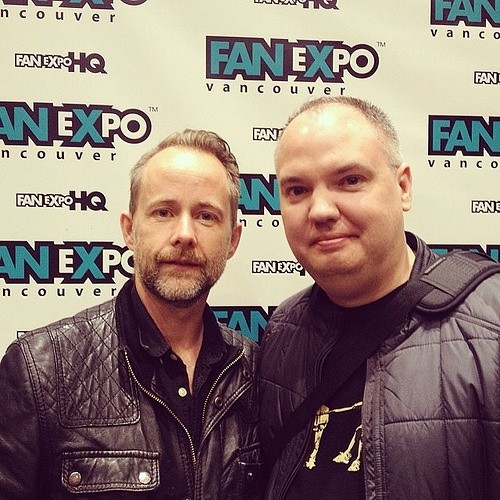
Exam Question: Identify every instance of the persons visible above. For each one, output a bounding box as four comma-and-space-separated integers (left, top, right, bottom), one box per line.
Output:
1, 129, 260, 500
258, 95, 500, 500
